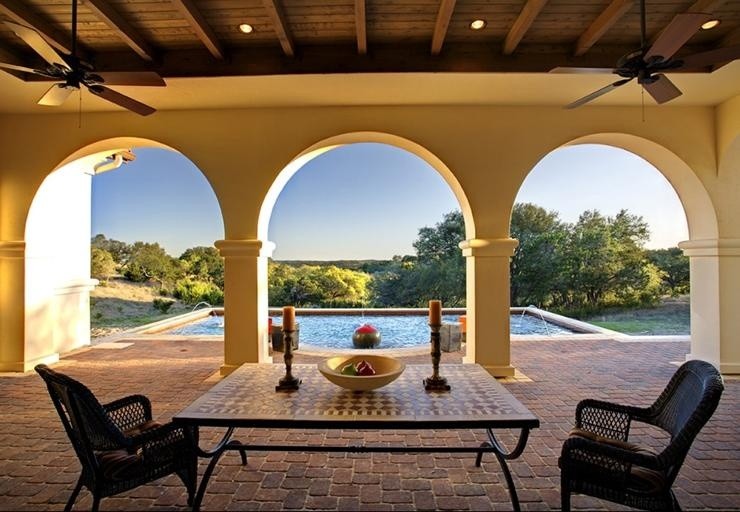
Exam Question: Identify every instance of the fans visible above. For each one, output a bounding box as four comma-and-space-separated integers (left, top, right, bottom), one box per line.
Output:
546, 0, 740, 120
0, 1, 168, 120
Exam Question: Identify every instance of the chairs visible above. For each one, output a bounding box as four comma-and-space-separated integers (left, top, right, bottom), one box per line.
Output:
558, 358, 725, 511
31, 364, 201, 512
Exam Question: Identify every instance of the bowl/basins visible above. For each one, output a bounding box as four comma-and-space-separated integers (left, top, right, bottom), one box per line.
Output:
315, 351, 406, 393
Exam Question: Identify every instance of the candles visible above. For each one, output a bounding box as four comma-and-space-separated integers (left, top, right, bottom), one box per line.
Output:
428, 300, 442, 325
282, 306, 296, 331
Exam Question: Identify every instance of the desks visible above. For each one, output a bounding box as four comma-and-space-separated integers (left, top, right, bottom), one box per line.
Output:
173, 362, 541, 512
